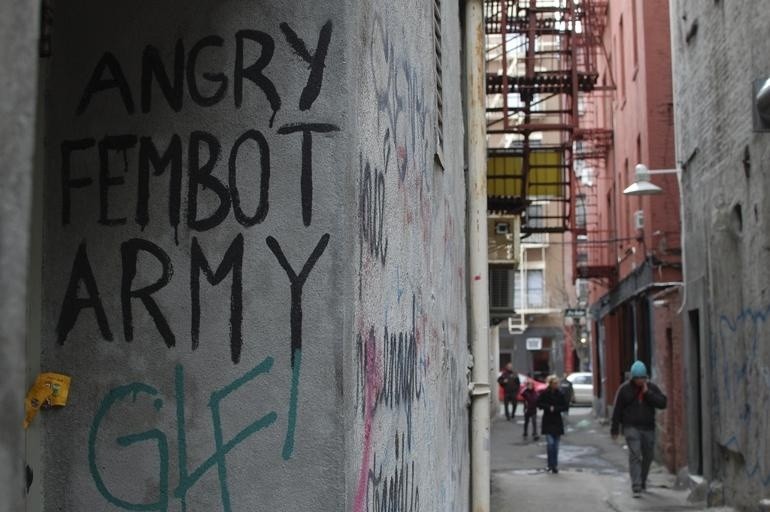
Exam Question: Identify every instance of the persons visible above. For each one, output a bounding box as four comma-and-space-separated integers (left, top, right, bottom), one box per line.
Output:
537, 374, 568, 473
559, 371, 576, 414
498, 363, 520, 421
520, 380, 540, 442
609, 360, 669, 496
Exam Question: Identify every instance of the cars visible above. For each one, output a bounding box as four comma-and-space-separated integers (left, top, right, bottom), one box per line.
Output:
567, 371, 594, 406
498, 370, 547, 400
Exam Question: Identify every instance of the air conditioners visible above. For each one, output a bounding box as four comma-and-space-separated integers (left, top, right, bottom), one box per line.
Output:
634, 210, 644, 230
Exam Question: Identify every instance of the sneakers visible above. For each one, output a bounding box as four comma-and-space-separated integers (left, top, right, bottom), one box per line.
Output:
633, 482, 646, 492
546, 464, 558, 473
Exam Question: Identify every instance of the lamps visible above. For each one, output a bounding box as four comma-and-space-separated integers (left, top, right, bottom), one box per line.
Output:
621, 160, 688, 196
652, 300, 670, 309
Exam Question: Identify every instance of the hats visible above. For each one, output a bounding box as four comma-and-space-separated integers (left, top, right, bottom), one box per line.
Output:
630, 361, 648, 376
